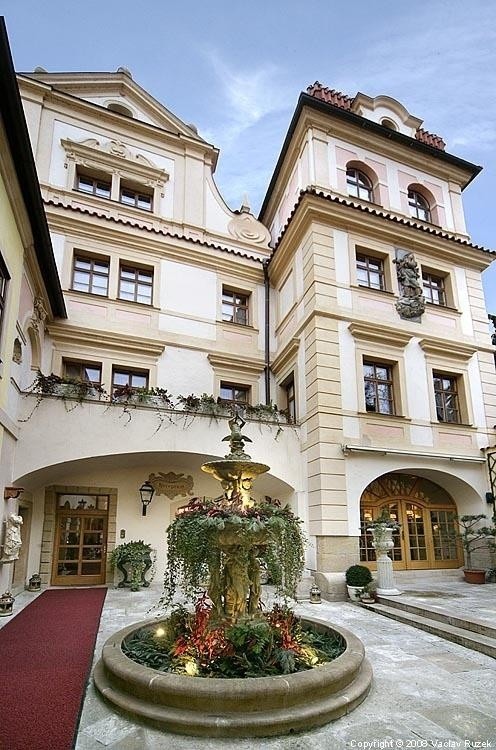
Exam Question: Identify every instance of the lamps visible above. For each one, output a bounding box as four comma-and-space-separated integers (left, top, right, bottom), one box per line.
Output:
137, 481, 154, 517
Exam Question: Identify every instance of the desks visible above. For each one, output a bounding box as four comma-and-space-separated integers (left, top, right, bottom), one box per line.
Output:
115, 560, 151, 587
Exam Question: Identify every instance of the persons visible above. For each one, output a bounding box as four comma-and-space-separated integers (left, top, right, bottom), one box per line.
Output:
397, 253, 422, 296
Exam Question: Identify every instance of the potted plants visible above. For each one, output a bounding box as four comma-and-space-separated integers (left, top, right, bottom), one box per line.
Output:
345, 565, 373, 602
433, 514, 495, 584
